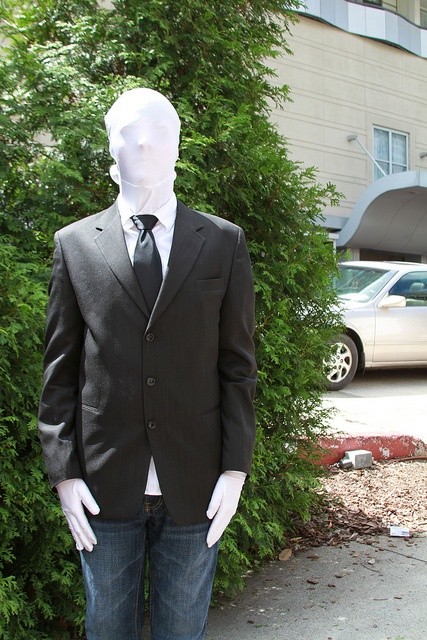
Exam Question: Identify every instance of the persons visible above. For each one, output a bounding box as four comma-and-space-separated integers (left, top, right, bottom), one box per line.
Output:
37, 87, 257, 640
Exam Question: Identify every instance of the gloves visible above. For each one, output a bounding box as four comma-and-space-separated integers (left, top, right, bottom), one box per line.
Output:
56, 478, 100, 553
206, 469, 247, 548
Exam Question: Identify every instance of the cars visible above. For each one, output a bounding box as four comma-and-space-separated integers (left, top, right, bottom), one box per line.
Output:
293, 259, 426, 391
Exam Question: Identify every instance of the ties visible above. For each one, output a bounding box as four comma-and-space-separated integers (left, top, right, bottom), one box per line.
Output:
131, 214, 162, 315
